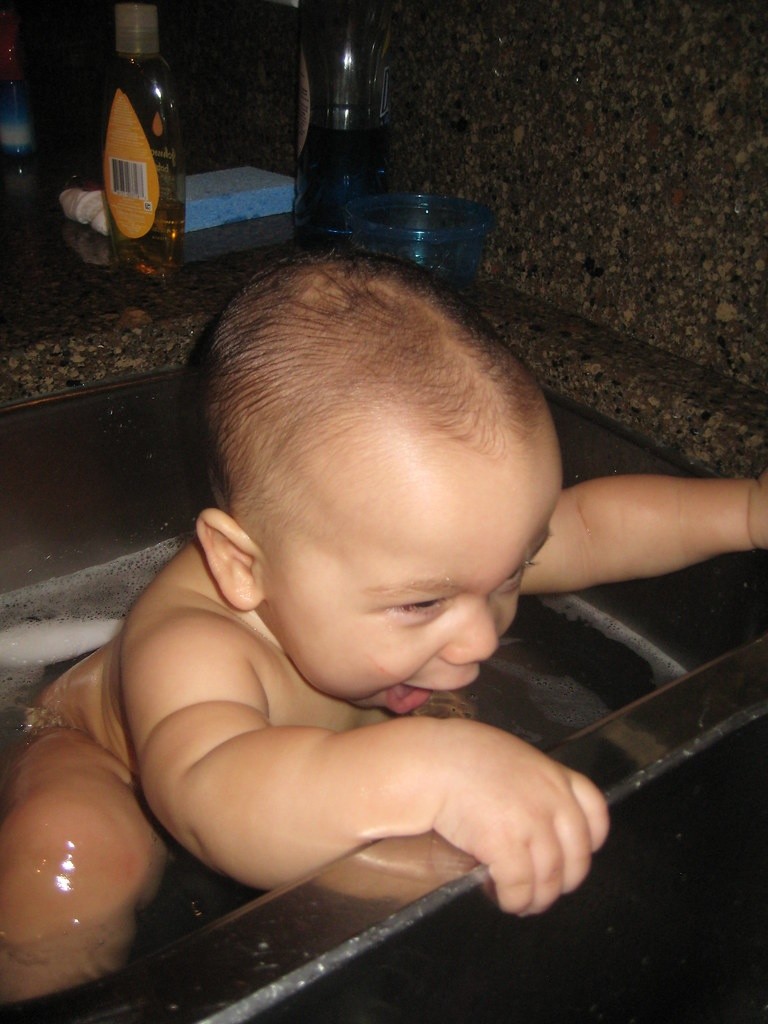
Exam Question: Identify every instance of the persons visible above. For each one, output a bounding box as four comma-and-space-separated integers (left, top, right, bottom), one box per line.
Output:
0, 242, 768, 938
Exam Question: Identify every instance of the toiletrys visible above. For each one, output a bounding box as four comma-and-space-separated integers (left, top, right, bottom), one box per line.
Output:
99, 3, 190, 252
293, 1, 408, 235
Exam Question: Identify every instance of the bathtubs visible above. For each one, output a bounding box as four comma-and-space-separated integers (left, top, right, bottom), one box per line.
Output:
0, 360, 768, 1024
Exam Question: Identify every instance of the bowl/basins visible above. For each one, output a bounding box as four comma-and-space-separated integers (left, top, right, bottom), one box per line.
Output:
344, 194, 496, 289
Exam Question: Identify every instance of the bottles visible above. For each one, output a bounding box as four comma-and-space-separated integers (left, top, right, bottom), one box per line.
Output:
101, 4, 186, 282
